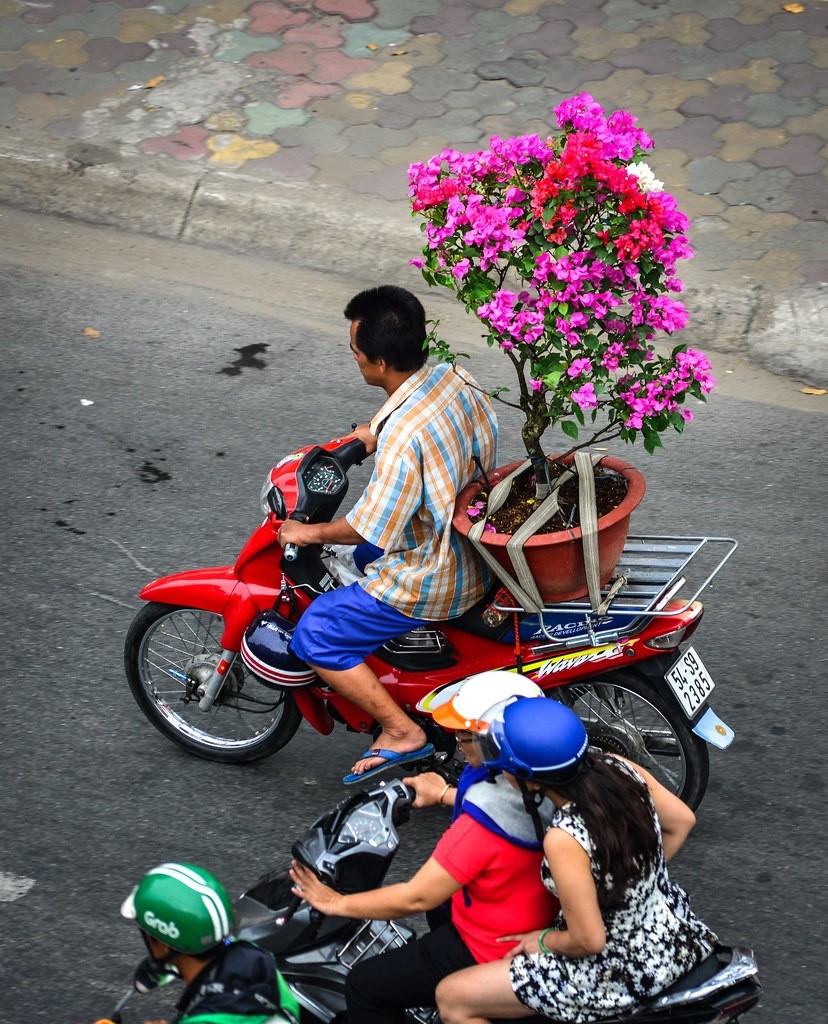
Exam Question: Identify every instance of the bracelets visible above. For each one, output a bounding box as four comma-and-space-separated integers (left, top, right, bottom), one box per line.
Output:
440, 783, 452, 804
539, 928, 558, 956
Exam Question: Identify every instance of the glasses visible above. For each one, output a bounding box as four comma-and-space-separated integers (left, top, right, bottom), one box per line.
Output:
453, 731, 486, 747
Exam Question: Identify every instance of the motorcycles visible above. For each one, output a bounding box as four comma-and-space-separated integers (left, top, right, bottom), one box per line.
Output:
231, 781, 766, 1024
121, 428, 741, 833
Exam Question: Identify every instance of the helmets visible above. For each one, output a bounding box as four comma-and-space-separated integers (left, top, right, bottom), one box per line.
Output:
482, 698, 590, 784
432, 671, 545, 735
119, 861, 233, 953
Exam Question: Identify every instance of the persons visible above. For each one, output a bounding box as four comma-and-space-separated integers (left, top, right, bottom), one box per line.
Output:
435, 696, 695, 1024
290, 669, 560, 1024
275, 284, 500, 786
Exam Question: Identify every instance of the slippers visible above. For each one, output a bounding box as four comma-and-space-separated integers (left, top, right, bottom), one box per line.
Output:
343, 742, 436, 785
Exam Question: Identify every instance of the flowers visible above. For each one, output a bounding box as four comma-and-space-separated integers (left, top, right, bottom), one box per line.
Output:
409, 93, 716, 509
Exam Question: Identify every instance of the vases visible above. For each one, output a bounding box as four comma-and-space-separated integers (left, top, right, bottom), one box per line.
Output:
457, 451, 648, 606
